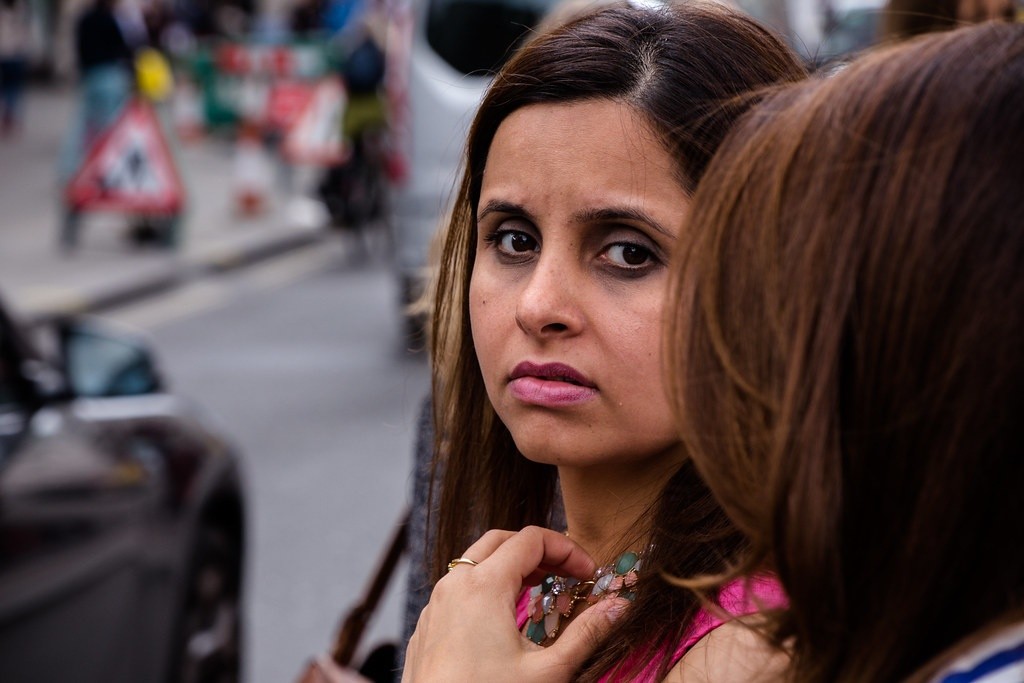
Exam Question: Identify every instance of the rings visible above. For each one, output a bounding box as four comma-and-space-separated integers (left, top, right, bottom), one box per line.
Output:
447, 558, 479, 571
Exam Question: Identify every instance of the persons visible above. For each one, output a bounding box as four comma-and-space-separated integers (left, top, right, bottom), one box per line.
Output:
654, 18, 1024, 683
389, 0, 812, 683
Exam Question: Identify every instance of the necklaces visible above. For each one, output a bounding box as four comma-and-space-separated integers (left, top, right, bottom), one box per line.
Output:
522, 524, 655, 645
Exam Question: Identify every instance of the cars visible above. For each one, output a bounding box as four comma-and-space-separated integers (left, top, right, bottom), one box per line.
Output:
0, 283, 251, 683
377, 0, 835, 344
